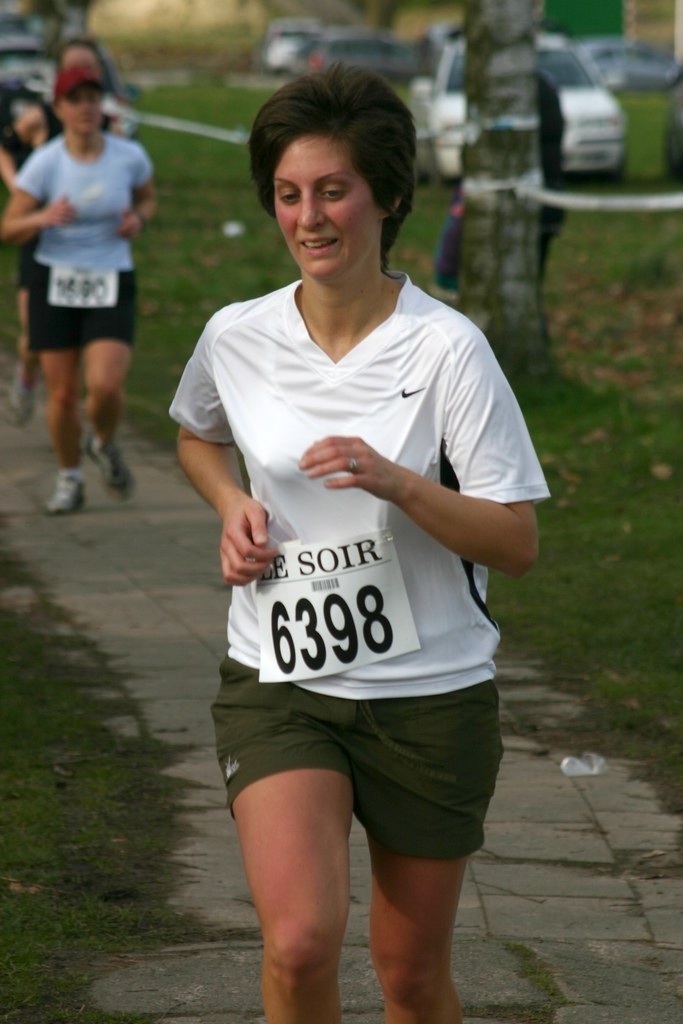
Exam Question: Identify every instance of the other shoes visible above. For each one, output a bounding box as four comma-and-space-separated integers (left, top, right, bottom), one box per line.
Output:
8, 365, 35, 426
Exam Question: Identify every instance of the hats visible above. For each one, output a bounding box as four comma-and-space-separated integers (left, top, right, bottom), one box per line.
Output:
54, 68, 103, 97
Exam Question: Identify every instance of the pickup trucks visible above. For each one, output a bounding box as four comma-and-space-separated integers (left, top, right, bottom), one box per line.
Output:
410, 37, 627, 192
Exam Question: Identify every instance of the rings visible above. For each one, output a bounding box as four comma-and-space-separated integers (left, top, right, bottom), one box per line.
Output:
349, 457, 356, 470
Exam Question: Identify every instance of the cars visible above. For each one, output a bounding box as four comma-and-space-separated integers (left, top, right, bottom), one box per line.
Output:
583, 35, 683, 95
264, 16, 430, 86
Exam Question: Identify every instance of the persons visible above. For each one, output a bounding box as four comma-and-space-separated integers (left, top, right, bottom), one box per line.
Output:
434, 67, 564, 289
167, 68, 552, 1024
0, 39, 160, 515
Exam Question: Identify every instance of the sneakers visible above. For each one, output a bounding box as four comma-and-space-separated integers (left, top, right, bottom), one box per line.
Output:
45, 475, 85, 513
83, 426, 133, 498
